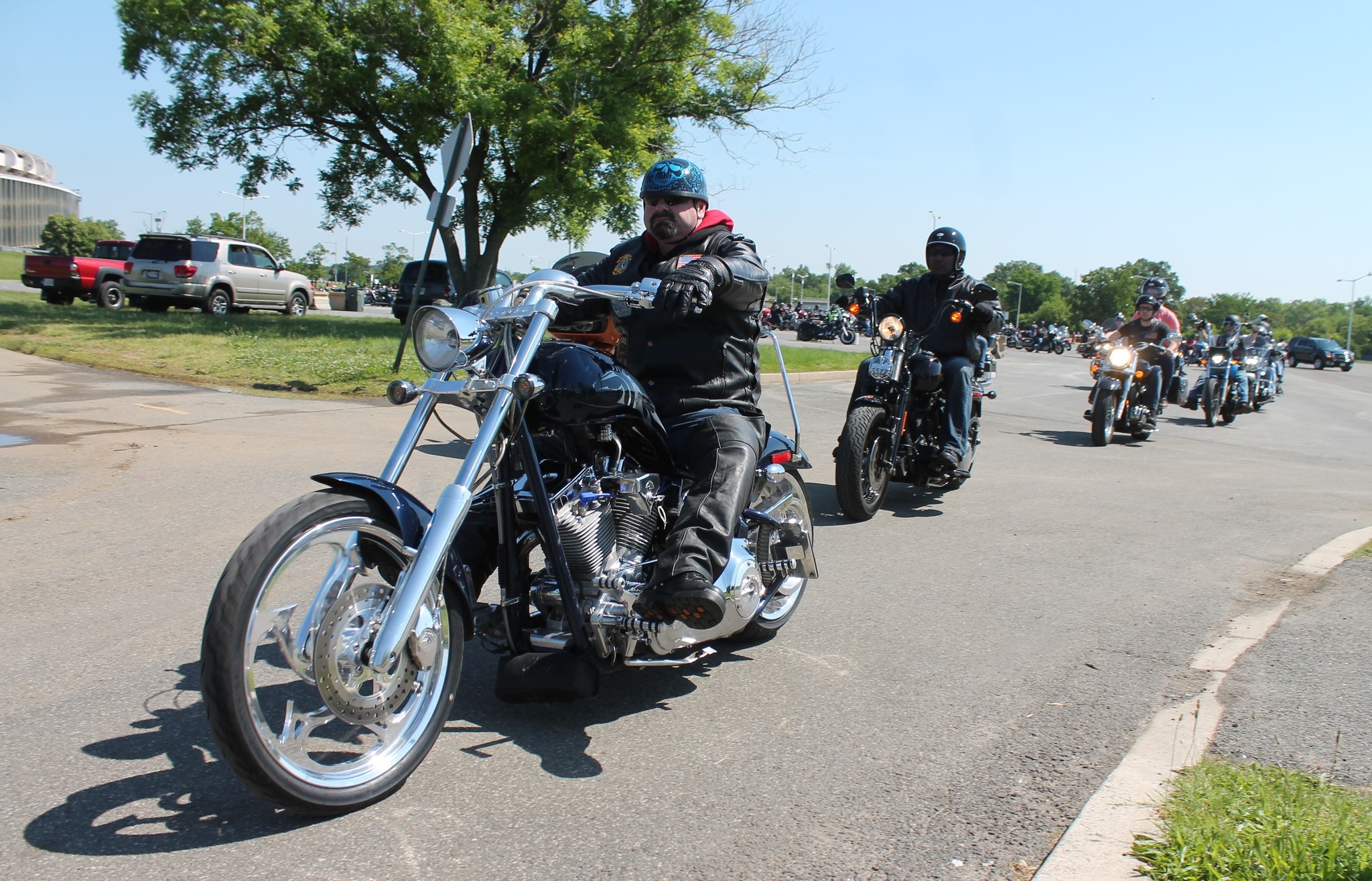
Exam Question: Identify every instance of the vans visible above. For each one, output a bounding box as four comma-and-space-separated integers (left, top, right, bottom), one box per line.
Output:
393, 259, 513, 325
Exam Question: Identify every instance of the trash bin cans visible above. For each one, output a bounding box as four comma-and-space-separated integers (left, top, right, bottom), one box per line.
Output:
345, 287, 366, 312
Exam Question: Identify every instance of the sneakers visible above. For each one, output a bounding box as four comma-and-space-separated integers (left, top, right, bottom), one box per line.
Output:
631, 570, 727, 630
937, 448, 961, 469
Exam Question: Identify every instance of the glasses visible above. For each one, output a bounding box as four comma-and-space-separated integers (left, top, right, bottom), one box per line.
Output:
643, 195, 694, 206
928, 247, 956, 257
1138, 306, 1155, 311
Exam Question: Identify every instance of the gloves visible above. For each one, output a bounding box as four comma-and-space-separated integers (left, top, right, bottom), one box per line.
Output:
835, 293, 855, 309
970, 302, 994, 324
652, 260, 723, 324
456, 289, 483, 310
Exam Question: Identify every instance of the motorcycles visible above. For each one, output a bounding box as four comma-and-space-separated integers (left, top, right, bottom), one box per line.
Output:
760, 306, 1289, 428
319, 287, 399, 308
199, 269, 822, 818
1074, 319, 1188, 447
834, 273, 998, 522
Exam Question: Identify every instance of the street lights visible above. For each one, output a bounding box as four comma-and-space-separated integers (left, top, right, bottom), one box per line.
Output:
824, 243, 837, 312
133, 209, 168, 234
1008, 281, 1023, 328
219, 190, 270, 240
757, 253, 775, 267
797, 274, 809, 305
1336, 272, 1372, 350
324, 241, 338, 268
399, 229, 430, 260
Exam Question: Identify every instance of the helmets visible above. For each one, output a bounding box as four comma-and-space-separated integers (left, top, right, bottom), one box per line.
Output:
1135, 294, 1161, 310
1140, 276, 1169, 304
639, 158, 709, 211
925, 227, 966, 270
1113, 311, 1126, 324
1223, 314, 1241, 326
1256, 324, 1269, 336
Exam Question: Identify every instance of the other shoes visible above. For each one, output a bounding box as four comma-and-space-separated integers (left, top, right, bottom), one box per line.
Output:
1146, 414, 1157, 426
1180, 401, 1198, 410
1265, 395, 1274, 400
1083, 409, 1094, 420
1276, 379, 1283, 384
1159, 398, 1169, 408
1231, 402, 1254, 415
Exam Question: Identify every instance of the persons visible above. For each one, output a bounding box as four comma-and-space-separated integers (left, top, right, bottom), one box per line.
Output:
378, 160, 771, 630
771, 299, 1059, 355
1083, 277, 1181, 427
832, 227, 1005, 468
1180, 315, 1289, 415
311, 280, 399, 307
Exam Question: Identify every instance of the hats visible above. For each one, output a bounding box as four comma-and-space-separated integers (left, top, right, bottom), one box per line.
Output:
1268, 331, 1274, 337
1280, 338, 1286, 340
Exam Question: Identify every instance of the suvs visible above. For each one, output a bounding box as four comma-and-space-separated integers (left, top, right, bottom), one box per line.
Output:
119, 229, 315, 317
1288, 336, 1356, 372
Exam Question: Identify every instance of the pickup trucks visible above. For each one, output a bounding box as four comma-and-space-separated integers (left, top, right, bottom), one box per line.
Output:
19, 239, 139, 314
